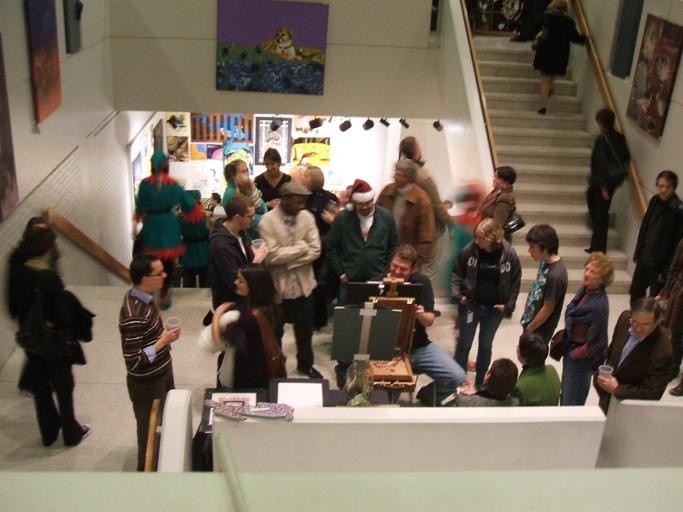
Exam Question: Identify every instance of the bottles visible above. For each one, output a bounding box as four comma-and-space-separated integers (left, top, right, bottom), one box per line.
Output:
326, 199, 337, 216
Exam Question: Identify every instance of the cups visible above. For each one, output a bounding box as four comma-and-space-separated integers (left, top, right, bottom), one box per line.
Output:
251, 239, 262, 249
166, 317, 180, 331
599, 364, 613, 378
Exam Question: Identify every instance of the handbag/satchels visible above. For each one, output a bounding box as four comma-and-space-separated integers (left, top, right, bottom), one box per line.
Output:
550, 329, 566, 360
532, 31, 543, 49
505, 218, 524, 232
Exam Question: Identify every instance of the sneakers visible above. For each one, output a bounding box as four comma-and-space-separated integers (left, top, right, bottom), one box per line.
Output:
82, 424, 90, 437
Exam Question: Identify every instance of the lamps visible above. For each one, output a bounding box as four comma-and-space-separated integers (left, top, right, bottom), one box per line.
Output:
270, 119, 443, 131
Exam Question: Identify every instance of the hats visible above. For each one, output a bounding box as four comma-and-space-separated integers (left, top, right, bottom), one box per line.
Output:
280, 182, 312, 196
346, 179, 374, 211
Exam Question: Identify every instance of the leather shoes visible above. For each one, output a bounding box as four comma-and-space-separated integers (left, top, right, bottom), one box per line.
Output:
297, 367, 322, 379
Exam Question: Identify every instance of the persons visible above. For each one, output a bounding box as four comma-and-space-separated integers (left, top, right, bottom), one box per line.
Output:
130, 136, 567, 405
584, 108, 631, 255
6, 217, 64, 320
593, 297, 675, 416
557, 250, 615, 405
464, 1, 548, 39
17, 230, 96, 447
533, 0, 588, 115
656, 240, 683, 396
629, 170, 682, 305
118, 255, 183, 472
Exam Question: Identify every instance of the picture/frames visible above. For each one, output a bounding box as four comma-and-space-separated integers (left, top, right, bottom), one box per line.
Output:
201, 387, 266, 436
268, 378, 330, 407
255, 117, 292, 165
